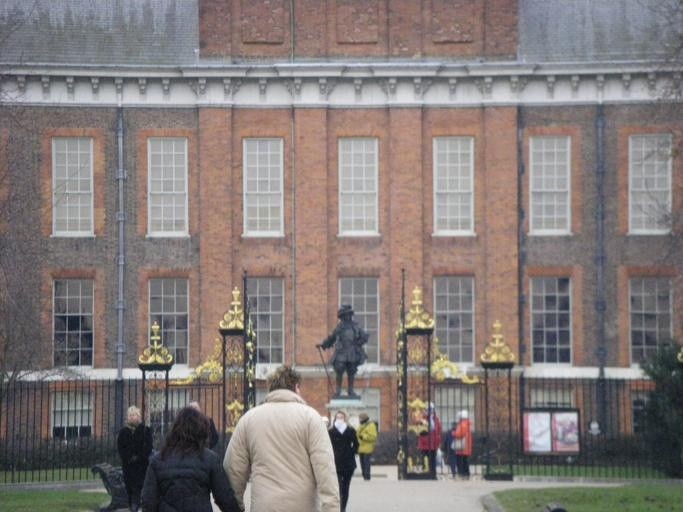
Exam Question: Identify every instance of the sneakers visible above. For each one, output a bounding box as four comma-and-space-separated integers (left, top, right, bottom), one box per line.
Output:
361, 471, 470, 481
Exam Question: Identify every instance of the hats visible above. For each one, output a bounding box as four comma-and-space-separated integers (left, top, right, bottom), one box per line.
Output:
456, 410, 468, 418
424, 400, 435, 408
358, 411, 368, 422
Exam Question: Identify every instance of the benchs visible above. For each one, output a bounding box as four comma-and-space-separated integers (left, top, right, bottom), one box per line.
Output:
91, 461, 130, 512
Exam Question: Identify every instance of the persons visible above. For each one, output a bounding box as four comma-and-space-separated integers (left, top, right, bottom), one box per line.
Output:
321, 305, 370, 400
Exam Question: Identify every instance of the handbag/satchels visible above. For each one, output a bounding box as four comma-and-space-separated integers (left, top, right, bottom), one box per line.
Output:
450, 438, 464, 450
